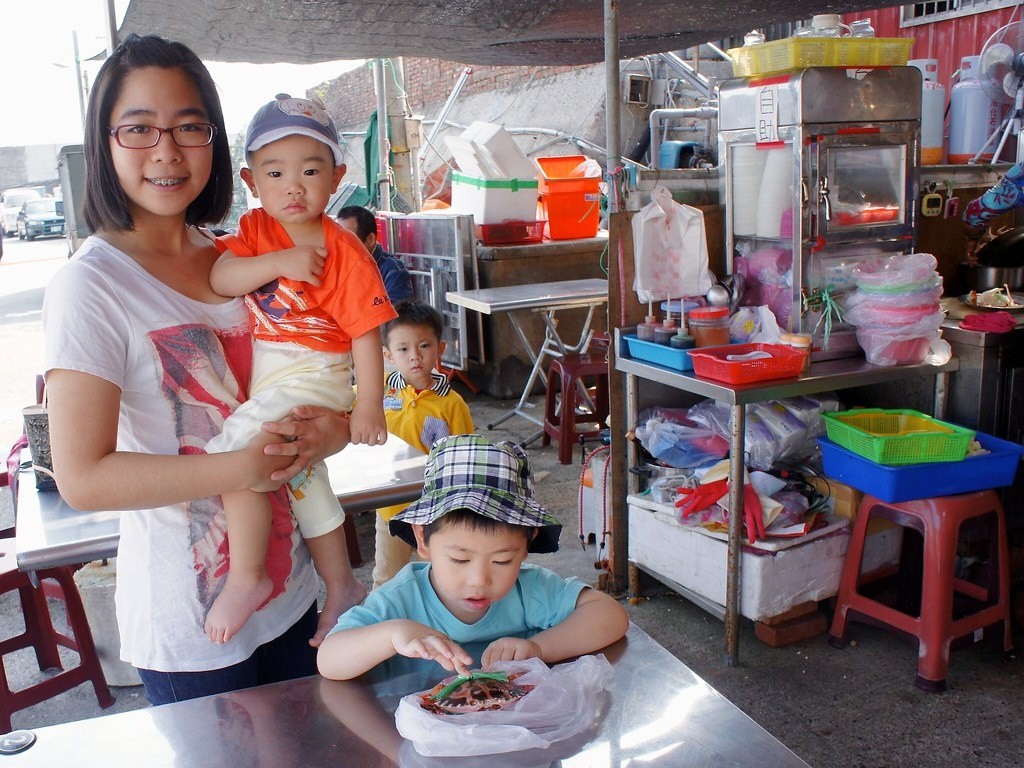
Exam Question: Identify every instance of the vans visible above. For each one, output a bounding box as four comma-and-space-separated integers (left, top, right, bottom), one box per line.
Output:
0, 188, 42, 238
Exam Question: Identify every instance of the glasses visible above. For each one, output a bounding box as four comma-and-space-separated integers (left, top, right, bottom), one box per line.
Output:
102, 123, 218, 149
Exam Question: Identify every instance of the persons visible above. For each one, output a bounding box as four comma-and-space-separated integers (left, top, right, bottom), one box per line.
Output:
155, 637, 628, 768
352, 304, 474, 590
44, 36, 349, 706
317, 434, 629, 680
204, 95, 399, 647
338, 205, 413, 304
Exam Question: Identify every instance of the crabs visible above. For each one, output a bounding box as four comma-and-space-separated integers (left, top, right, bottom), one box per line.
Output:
417, 671, 537, 725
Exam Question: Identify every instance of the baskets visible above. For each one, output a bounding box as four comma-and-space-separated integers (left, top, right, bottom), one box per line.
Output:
687, 343, 809, 385
474, 220, 547, 245
726, 38, 914, 76
834, 207, 898, 225
821, 407, 976, 467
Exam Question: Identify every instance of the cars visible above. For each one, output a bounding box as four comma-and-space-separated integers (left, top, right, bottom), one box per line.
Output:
16, 198, 67, 241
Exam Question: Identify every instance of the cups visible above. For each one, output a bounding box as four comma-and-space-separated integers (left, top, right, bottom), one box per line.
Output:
811, 14, 854, 38
23, 403, 57, 490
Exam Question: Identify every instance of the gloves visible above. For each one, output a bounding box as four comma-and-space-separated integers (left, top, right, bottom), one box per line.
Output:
742, 482, 765, 544
676, 480, 728, 519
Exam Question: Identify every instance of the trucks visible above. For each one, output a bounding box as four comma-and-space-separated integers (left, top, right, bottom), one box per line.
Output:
56, 144, 95, 260
25, 185, 63, 199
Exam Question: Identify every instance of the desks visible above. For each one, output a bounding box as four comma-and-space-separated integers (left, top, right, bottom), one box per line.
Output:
444, 279, 609, 448
613, 326, 961, 665
0, 621, 812, 768
17, 430, 428, 570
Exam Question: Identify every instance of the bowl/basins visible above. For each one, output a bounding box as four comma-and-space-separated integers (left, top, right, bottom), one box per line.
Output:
852, 253, 942, 366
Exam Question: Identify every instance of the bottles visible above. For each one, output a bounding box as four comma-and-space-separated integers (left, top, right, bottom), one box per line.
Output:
637, 292, 695, 348
742, 19, 875, 46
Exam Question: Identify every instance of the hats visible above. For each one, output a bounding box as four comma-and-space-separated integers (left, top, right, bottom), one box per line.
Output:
244, 93, 344, 166
388, 434, 562, 554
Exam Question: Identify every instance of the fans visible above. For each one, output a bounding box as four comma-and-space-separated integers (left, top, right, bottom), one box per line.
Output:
978, 21, 1024, 137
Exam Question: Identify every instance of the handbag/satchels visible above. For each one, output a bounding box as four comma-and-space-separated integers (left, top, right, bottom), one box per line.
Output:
633, 406, 731, 467
21, 385, 57, 490
394, 654, 615, 757
730, 304, 785, 344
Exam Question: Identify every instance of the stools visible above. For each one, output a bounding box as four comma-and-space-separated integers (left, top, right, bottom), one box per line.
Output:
540, 332, 613, 464
0, 470, 361, 734
828, 490, 1015, 691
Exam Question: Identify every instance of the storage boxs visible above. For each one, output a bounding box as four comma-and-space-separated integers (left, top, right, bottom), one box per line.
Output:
621, 335, 1023, 621
324, 121, 603, 271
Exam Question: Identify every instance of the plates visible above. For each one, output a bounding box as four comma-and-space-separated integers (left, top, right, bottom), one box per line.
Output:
958, 293, 1023, 313
